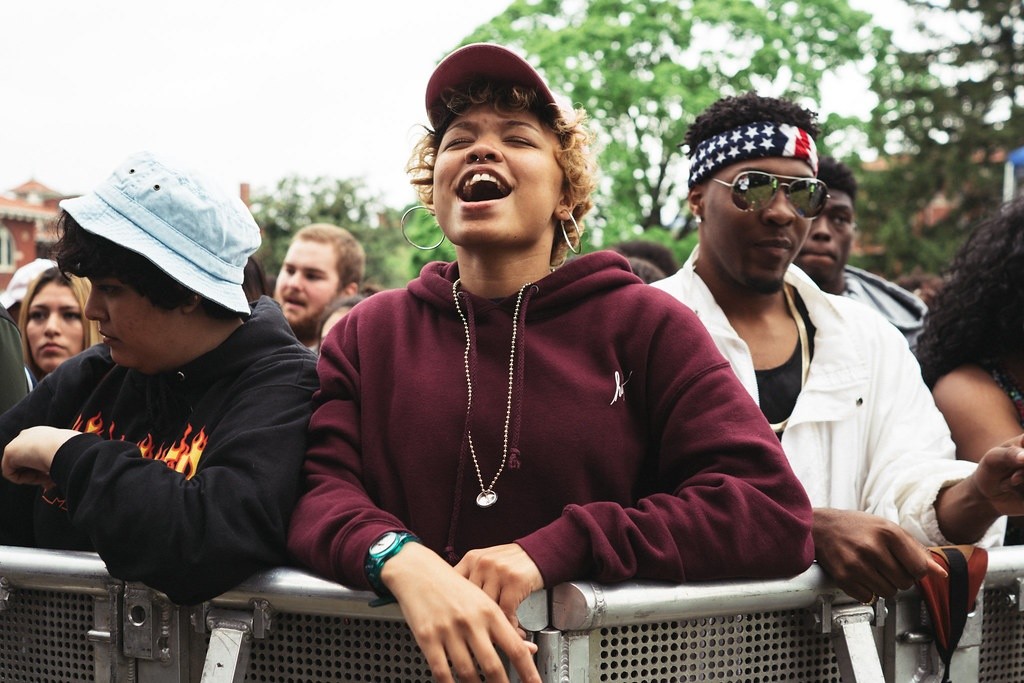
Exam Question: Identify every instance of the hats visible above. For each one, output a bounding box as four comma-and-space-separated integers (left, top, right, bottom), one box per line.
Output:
59, 153, 262, 314
425, 42, 563, 132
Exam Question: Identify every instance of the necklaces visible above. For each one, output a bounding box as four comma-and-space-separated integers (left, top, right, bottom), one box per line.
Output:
453, 275, 533, 508
765, 280, 808, 430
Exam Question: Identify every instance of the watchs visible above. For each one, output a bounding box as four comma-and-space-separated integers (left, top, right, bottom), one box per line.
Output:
364, 532, 421, 607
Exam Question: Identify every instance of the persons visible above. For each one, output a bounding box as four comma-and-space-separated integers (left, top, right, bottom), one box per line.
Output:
286, 42, 815, 683
610, 241, 679, 287
244, 221, 385, 357
912, 194, 1024, 546
645, 92, 1024, 606
795, 155, 929, 354
0, 259, 104, 419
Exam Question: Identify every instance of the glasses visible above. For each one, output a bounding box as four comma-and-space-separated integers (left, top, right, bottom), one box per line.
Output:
711, 170, 833, 220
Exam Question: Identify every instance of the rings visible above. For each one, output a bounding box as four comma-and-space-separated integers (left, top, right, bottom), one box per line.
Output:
862, 592, 876, 606
